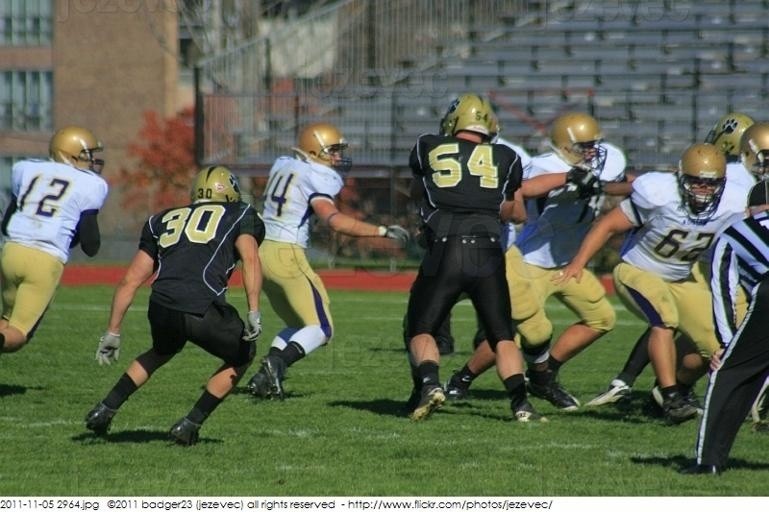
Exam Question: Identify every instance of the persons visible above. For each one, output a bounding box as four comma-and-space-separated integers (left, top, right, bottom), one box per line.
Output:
84, 164, 266, 446
417, 130, 532, 355
551, 141, 748, 425
407, 94, 549, 422
0, 127, 110, 354
676, 122, 769, 415
247, 123, 411, 402
685, 177, 769, 474
444, 111, 627, 411
588, 110, 749, 406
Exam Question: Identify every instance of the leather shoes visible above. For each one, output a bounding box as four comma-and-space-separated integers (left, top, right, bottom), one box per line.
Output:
681, 462, 723, 477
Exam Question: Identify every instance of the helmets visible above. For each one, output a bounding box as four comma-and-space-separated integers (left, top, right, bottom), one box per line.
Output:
704, 110, 756, 161
189, 164, 243, 204
740, 120, 769, 181
48, 126, 105, 175
439, 92, 500, 144
298, 122, 352, 174
551, 110, 608, 170
676, 141, 727, 224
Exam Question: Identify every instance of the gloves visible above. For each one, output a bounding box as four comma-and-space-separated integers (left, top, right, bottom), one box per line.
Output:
565, 164, 602, 195
94, 331, 121, 367
240, 311, 263, 342
378, 222, 412, 244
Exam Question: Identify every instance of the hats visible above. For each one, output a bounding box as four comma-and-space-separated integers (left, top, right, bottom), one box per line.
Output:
747, 179, 769, 206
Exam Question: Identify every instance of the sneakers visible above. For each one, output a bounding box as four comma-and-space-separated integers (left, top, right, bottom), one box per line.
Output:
661, 397, 700, 425
584, 379, 633, 408
410, 383, 445, 421
446, 369, 467, 401
169, 416, 202, 445
513, 401, 550, 424
650, 384, 706, 417
259, 354, 285, 402
524, 370, 582, 412
247, 371, 269, 400
84, 401, 118, 435
